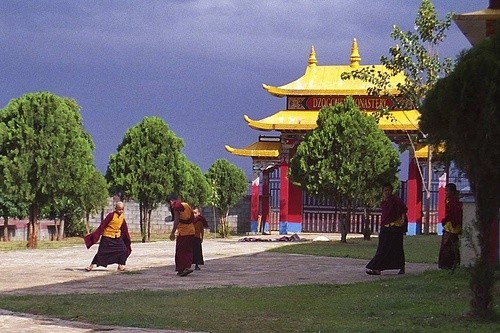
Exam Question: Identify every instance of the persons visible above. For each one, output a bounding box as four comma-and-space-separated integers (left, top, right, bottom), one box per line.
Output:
83, 201, 132, 271
364, 185, 408, 275
165, 195, 209, 276
438, 183, 463, 275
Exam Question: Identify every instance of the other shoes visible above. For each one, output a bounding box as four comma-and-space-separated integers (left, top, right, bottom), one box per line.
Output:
85, 267, 92, 271
397, 269, 405, 274
117, 268, 126, 272
194, 266, 200, 270
177, 271, 184, 275
180, 269, 193, 277
365, 270, 381, 276
